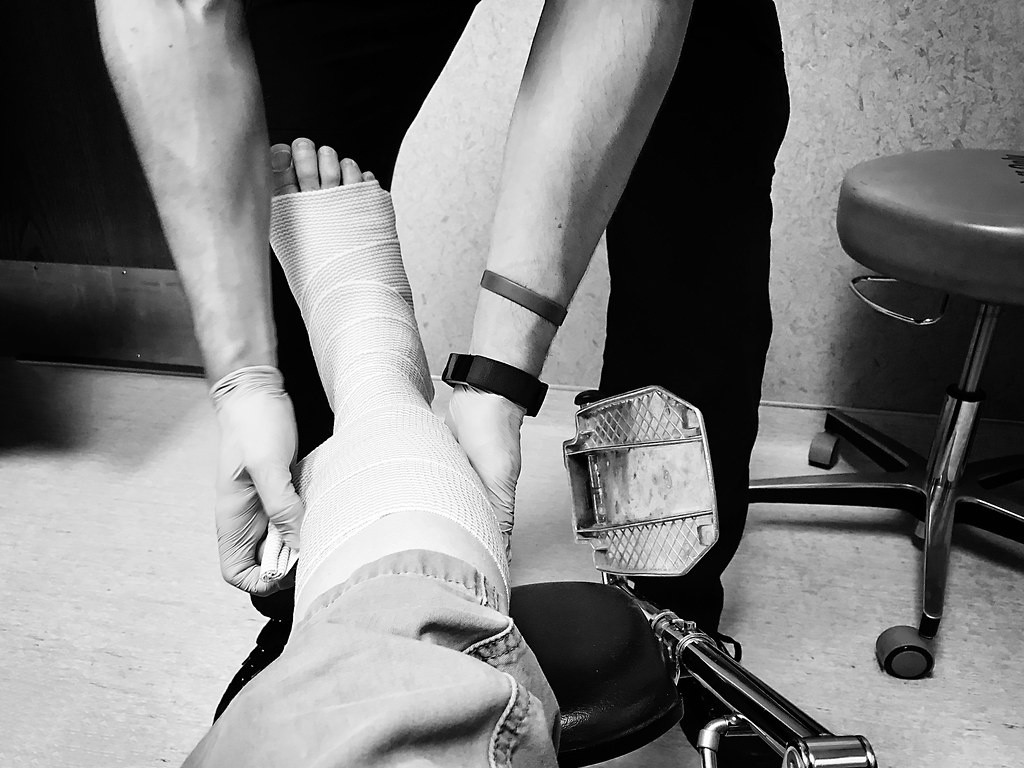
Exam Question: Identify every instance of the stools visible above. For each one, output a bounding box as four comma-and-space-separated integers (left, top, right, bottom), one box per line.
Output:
741, 144, 1024, 678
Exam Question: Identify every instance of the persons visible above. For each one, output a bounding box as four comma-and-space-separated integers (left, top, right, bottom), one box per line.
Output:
182, 137, 561, 768
96, 0, 791, 640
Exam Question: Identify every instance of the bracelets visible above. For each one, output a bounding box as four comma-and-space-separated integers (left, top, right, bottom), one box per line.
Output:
480, 269, 568, 327
441, 353, 549, 419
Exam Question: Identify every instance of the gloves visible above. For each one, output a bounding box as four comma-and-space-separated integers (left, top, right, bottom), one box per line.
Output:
208, 366, 306, 597
443, 380, 525, 566
